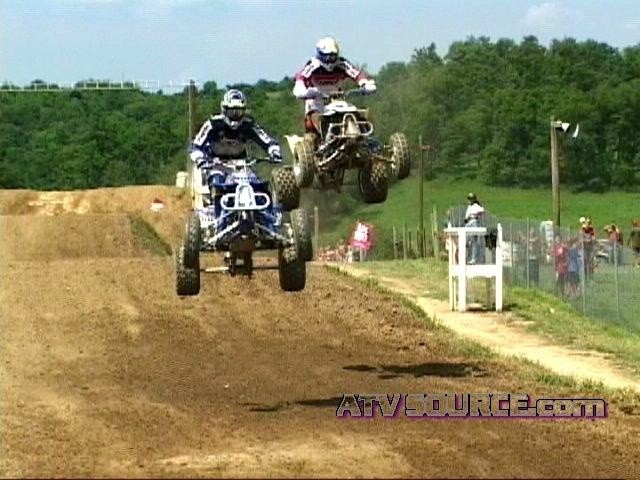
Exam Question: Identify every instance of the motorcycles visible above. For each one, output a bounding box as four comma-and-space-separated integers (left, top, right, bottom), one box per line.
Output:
171, 153, 312, 297
270, 80, 415, 213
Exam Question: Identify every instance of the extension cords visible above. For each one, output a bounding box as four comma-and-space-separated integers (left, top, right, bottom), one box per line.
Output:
561, 122, 580, 139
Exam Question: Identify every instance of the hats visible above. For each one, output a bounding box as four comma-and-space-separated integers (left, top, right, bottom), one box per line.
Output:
467, 193, 476, 200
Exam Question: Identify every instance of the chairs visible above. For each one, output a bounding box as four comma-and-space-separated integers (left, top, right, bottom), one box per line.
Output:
447, 222, 504, 313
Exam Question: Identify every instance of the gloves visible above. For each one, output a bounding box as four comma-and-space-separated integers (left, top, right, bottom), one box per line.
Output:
268, 150, 283, 164
306, 86, 321, 98
196, 158, 212, 170
361, 82, 377, 96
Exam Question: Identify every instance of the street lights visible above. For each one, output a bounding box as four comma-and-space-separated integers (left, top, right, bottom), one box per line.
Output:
548, 115, 570, 227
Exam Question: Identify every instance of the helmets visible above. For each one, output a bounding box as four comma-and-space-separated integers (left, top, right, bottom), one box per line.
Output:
221, 89, 248, 129
315, 36, 341, 72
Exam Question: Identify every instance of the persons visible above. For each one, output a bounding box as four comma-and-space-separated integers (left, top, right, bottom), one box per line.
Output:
516, 226, 541, 287
186, 88, 282, 226
579, 232, 600, 284
562, 236, 583, 296
463, 191, 487, 264
627, 220, 640, 259
604, 224, 624, 246
608, 224, 620, 266
581, 218, 596, 238
317, 237, 354, 262
291, 36, 377, 146
548, 233, 567, 297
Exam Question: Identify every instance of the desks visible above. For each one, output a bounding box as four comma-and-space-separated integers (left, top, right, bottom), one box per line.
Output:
443, 225, 489, 311
